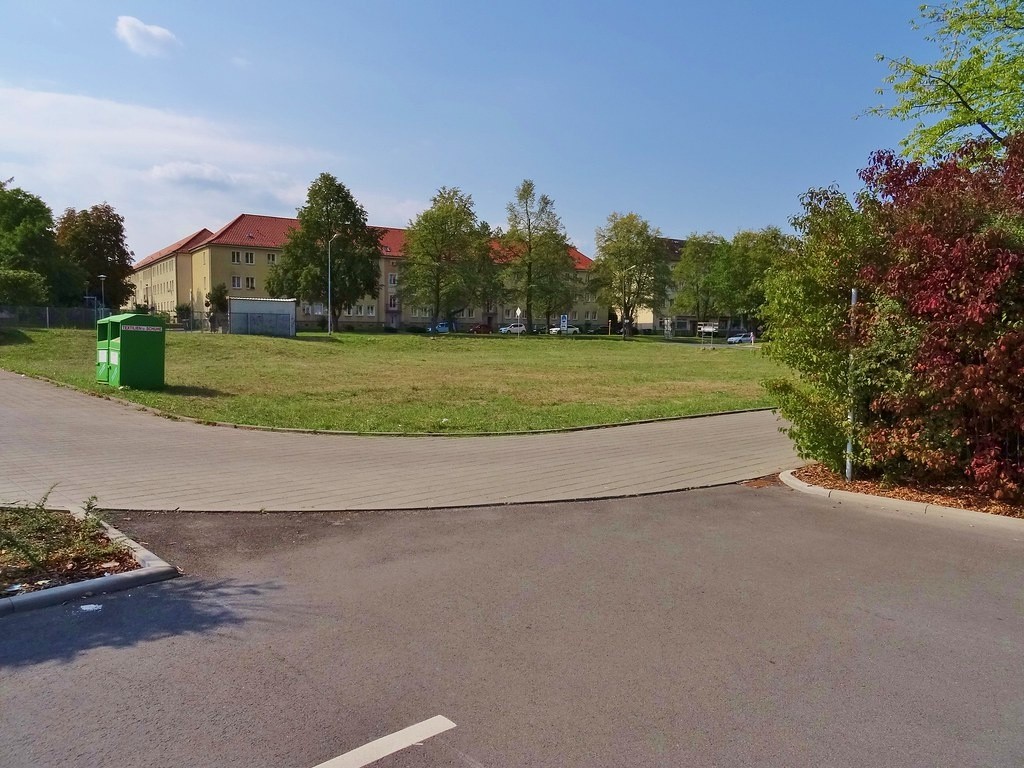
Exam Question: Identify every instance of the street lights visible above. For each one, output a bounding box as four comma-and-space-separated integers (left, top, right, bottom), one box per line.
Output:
328, 234, 338, 335
623, 265, 637, 340
83, 281, 89, 308
97, 274, 107, 319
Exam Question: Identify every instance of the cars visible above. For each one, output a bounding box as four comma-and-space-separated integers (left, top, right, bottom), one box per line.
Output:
533, 325, 557, 335
616, 327, 639, 335
427, 323, 456, 334
499, 323, 527, 335
466, 324, 493, 334
548, 325, 580, 335
697, 332, 717, 338
727, 332, 752, 344
587, 325, 615, 335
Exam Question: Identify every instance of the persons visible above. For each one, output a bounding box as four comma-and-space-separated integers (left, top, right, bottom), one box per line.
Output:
209, 313, 216, 333
749, 332, 755, 345
